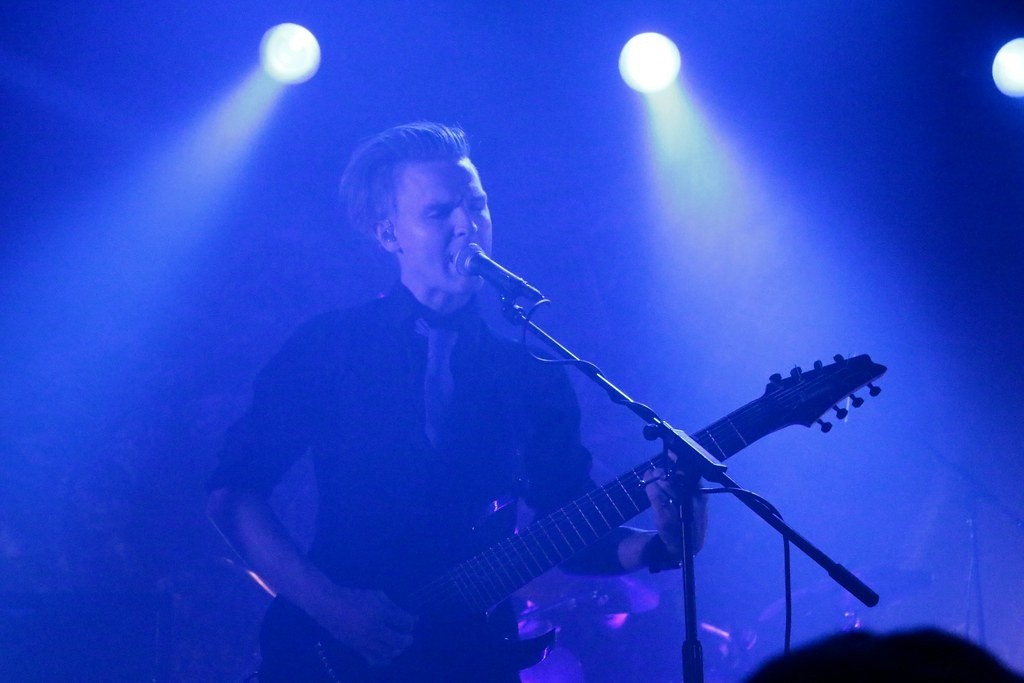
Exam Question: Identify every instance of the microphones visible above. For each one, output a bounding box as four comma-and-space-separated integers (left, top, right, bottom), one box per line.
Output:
451, 241, 544, 305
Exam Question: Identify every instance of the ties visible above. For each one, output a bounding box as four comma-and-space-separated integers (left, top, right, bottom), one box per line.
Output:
413, 316, 462, 455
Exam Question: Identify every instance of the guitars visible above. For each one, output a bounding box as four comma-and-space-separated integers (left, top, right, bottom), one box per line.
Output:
252, 351, 889, 683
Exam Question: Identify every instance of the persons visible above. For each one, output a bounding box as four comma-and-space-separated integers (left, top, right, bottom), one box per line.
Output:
198, 121, 713, 683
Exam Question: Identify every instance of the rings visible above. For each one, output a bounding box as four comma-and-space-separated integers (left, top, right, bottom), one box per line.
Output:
661, 498, 673, 508
